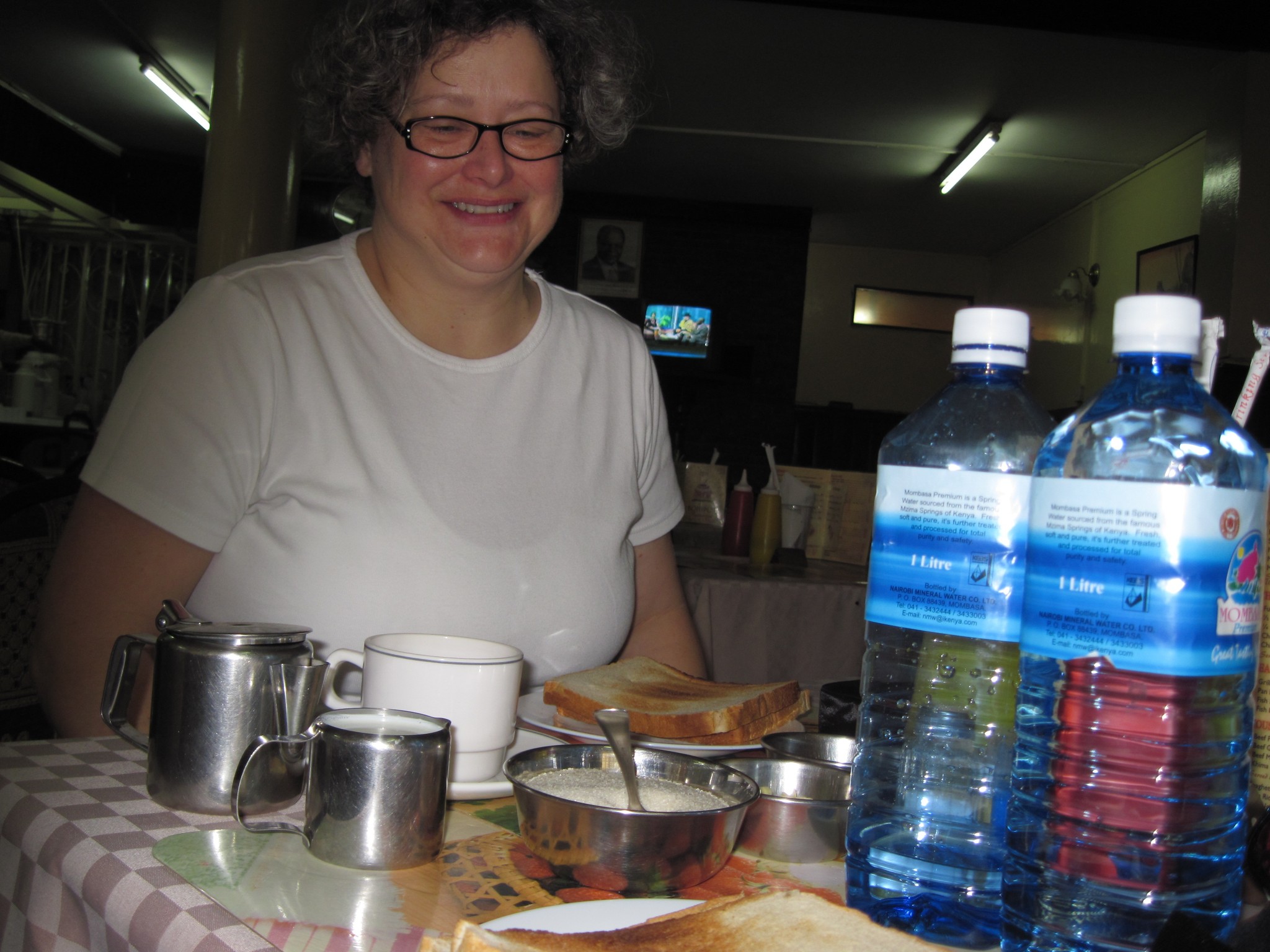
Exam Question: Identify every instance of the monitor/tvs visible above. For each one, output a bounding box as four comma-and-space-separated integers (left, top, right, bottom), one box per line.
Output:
640, 302, 716, 358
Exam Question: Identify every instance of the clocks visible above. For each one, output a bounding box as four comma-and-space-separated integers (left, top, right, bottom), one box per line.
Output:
332, 183, 373, 236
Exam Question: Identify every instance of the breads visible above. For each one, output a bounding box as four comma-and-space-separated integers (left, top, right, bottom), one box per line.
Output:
419, 888, 962, 952
543, 654, 811, 745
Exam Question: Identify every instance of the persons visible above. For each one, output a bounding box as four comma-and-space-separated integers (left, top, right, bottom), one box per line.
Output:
675, 313, 695, 332
644, 311, 657, 329
34, 0, 709, 739
677, 318, 708, 344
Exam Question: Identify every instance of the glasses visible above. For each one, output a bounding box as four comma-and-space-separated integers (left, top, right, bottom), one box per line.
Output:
379, 103, 571, 160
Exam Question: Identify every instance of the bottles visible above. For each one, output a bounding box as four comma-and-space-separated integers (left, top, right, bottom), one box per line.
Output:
721, 469, 755, 558
998, 294, 1270, 952
844, 310, 1057, 950
748, 471, 783, 565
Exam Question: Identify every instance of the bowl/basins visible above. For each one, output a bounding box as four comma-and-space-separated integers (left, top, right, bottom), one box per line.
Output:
502, 745, 761, 894
760, 731, 878, 777
714, 758, 872, 863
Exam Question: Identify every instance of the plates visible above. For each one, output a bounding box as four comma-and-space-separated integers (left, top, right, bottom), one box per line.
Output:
516, 690, 805, 760
446, 726, 572, 800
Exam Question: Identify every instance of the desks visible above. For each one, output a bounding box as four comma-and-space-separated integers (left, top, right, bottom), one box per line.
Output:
675, 537, 874, 728
0, 726, 852, 952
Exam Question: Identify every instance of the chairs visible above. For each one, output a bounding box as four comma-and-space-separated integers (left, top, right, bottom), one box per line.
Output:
4, 409, 95, 510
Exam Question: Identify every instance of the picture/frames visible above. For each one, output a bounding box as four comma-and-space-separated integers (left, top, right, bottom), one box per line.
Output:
574, 217, 644, 300
1135, 233, 1199, 297
850, 284, 974, 335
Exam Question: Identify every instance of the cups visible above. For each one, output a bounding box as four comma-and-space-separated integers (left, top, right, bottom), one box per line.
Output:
780, 503, 814, 566
99, 598, 331, 818
319, 632, 525, 783
818, 679, 913, 747
230, 707, 452, 872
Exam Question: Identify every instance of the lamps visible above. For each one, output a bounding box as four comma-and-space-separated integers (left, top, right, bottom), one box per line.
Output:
136, 40, 211, 133
936, 117, 1006, 195
1059, 263, 1101, 299
